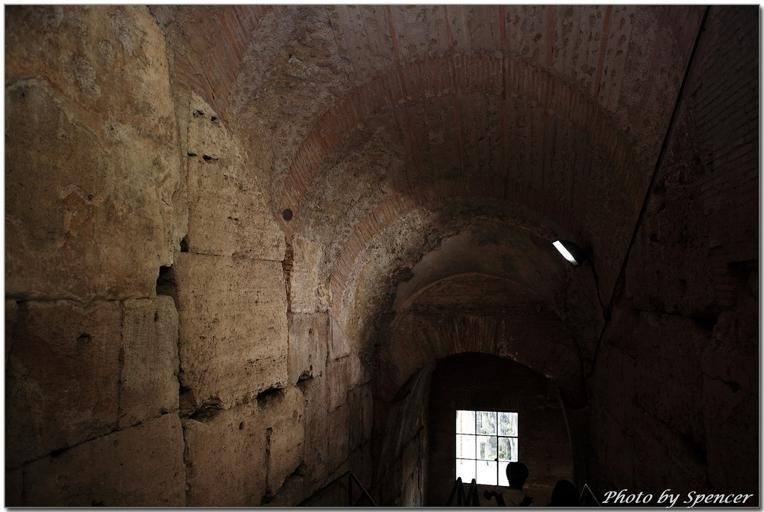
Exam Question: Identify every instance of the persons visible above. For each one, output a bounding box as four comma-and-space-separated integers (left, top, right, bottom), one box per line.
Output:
490, 462, 537, 507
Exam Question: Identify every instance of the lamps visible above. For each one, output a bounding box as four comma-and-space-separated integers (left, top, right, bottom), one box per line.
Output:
552, 239, 594, 268
